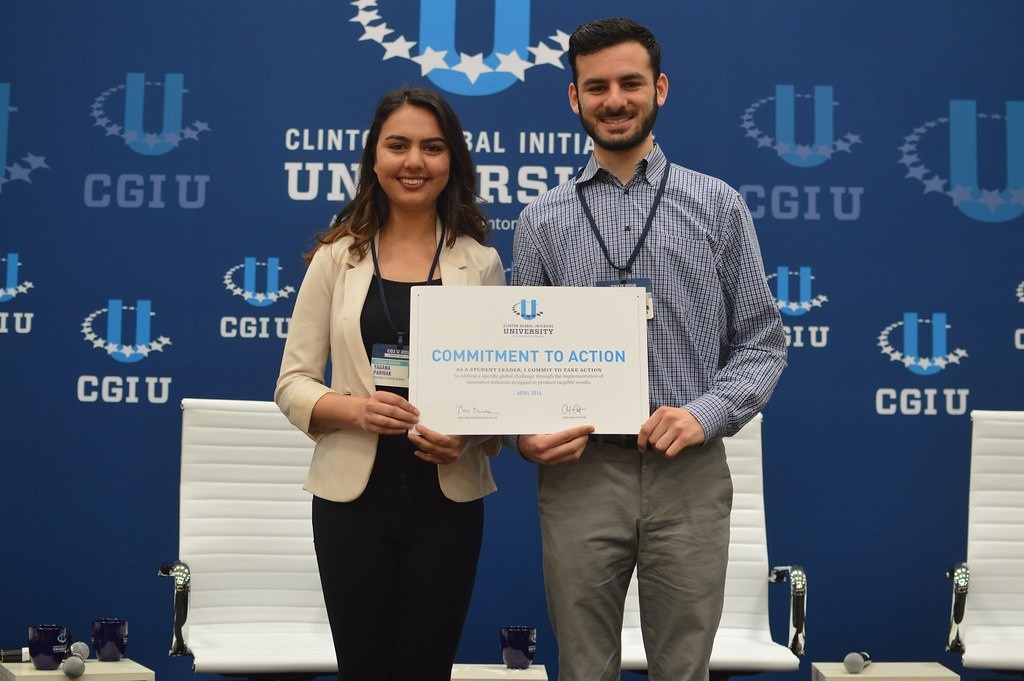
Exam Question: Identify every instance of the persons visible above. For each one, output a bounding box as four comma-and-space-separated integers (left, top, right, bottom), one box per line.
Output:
509, 19, 787, 681
274, 88, 507, 681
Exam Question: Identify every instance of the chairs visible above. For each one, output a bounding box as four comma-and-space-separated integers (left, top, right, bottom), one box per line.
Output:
618, 412, 807, 681
158, 397, 339, 681
945, 409, 1024, 679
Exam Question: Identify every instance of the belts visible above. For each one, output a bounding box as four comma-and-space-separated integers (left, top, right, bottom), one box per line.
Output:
588, 434, 638, 447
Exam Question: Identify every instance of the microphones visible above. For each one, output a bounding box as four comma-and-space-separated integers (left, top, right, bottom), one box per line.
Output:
0, 641, 90, 679
844, 651, 872, 674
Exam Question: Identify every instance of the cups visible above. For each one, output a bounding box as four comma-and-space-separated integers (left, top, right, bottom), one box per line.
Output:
500, 627, 536, 670
29, 626, 72, 670
94, 620, 128, 662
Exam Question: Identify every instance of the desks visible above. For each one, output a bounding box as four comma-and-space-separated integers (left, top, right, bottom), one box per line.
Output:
810, 660, 962, 681
0, 658, 154, 681
